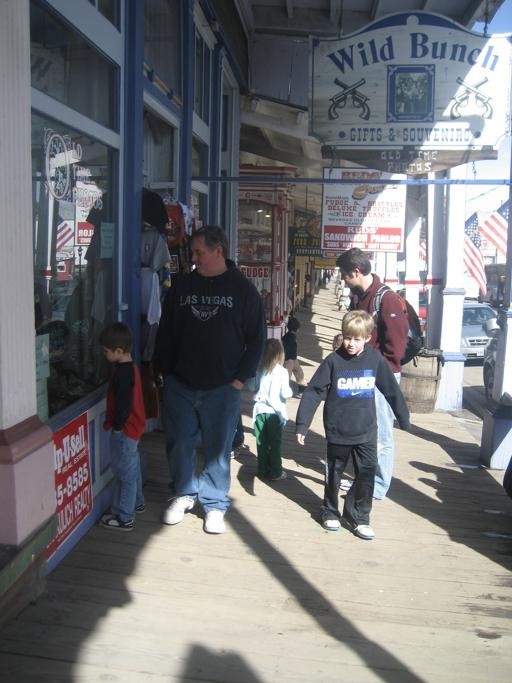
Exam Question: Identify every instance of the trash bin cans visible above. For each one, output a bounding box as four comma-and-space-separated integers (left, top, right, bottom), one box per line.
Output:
399, 347, 444, 414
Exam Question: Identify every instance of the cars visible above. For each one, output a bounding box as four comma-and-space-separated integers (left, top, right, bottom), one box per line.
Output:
460, 300, 500, 362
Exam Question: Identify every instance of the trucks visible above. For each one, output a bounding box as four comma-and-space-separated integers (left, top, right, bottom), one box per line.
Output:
396, 286, 428, 336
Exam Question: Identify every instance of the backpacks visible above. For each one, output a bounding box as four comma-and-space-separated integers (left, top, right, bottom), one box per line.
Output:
374, 284, 424, 365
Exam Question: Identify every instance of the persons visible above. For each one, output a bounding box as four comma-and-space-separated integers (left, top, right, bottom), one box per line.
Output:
283, 318, 308, 399
153, 225, 267, 534
252, 339, 293, 481
296, 311, 409, 539
335, 248, 409, 500
99, 321, 146, 530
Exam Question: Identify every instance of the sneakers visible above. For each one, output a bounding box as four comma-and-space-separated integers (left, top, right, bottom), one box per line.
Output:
162, 495, 194, 525
343, 517, 375, 539
321, 511, 341, 530
135, 505, 145, 514
203, 511, 225, 534
100, 516, 134, 531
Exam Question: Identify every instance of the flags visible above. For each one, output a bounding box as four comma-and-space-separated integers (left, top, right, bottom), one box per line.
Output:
480, 199, 509, 254
462, 213, 487, 296
56, 215, 74, 252
